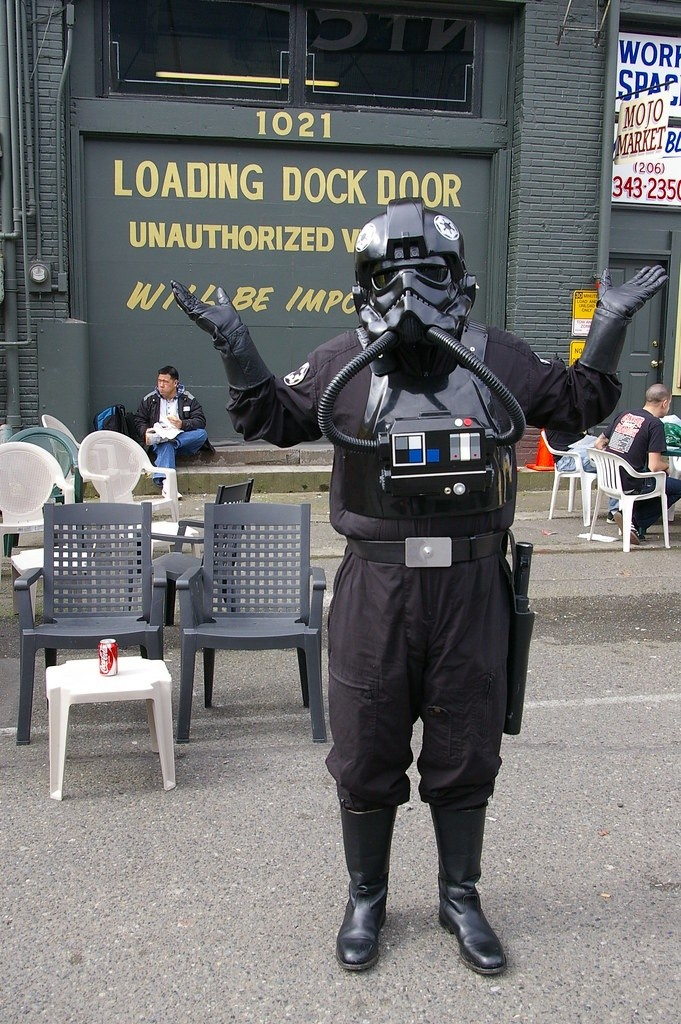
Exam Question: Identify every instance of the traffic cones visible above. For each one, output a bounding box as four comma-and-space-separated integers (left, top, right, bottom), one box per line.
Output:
526, 428, 554, 471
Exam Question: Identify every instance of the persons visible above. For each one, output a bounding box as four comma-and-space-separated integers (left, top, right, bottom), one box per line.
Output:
171, 197, 668, 975
540, 368, 622, 524
133, 366, 207, 500
594, 384, 681, 545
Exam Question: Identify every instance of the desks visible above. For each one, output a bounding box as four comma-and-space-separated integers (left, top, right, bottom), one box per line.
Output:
664, 449, 681, 480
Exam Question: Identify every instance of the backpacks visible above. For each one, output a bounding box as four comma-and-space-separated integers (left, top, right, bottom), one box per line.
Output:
92, 403, 130, 438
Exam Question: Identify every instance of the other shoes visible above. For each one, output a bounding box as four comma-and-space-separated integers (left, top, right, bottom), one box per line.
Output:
146, 432, 162, 446
161, 484, 183, 501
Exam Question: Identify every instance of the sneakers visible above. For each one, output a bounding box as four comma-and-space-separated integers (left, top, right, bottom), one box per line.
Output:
614, 512, 644, 546
606, 508, 621, 524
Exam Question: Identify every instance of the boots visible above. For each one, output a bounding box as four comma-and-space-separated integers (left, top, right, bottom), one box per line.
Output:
337, 799, 397, 971
428, 801, 507, 974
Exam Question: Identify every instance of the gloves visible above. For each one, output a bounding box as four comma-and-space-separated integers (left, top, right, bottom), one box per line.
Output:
578, 261, 671, 377
170, 277, 272, 389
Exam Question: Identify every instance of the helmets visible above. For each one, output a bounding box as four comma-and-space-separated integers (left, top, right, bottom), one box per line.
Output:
352, 195, 479, 376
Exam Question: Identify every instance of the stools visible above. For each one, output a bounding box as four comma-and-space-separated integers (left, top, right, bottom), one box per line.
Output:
41, 656, 176, 801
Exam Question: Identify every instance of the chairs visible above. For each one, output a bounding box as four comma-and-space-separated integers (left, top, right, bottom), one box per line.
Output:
0, 414, 327, 744
541, 429, 607, 526
585, 446, 671, 552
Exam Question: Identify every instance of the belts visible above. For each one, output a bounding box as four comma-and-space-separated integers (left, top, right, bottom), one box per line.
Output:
344, 530, 503, 569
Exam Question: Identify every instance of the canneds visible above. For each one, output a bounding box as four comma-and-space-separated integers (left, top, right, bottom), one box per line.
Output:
98, 638, 118, 677
